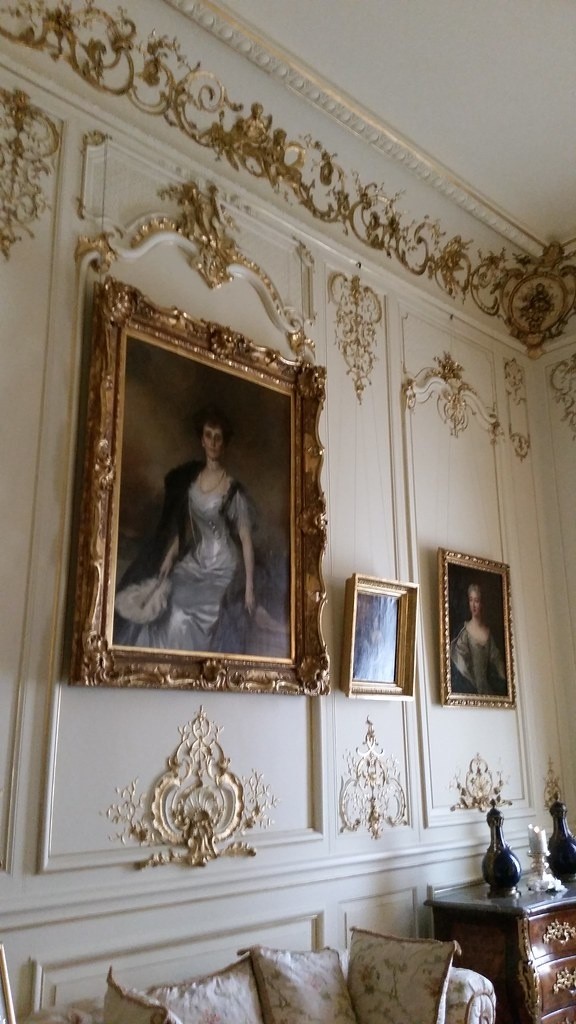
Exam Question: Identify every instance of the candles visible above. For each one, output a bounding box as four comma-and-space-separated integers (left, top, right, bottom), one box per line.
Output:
528, 825, 547, 854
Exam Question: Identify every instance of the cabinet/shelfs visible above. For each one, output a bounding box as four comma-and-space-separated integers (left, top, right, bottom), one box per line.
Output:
424, 900, 576, 1024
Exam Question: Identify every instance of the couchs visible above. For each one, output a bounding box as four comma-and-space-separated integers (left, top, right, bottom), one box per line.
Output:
12, 953, 495, 1024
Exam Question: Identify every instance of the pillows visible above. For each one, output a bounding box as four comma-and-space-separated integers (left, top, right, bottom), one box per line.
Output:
104, 966, 176, 1024
147, 956, 266, 1024
237, 944, 357, 1024
347, 927, 463, 1024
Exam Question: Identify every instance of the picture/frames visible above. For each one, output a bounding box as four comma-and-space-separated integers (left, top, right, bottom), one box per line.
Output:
341, 572, 419, 702
436, 546, 516, 709
58, 272, 331, 697
